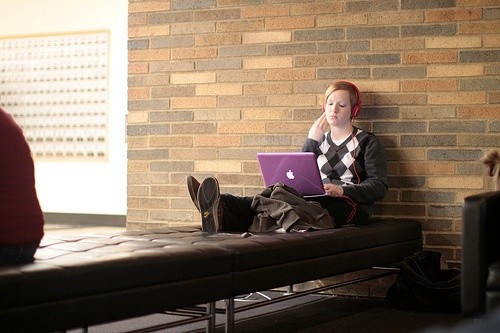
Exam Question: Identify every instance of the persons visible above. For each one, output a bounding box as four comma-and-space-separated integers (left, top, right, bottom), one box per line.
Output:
186, 82, 389, 236
0, 108, 45, 267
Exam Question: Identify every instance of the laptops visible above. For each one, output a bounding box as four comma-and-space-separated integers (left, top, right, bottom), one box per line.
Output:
257, 153, 338, 198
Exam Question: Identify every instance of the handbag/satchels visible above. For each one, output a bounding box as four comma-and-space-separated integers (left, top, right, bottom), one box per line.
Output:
384, 249, 462, 313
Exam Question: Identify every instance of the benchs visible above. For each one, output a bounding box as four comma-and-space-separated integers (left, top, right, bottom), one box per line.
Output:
0, 218, 423, 333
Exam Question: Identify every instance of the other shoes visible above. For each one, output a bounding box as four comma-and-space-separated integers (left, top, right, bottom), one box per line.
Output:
187, 175, 200, 212
200, 177, 221, 233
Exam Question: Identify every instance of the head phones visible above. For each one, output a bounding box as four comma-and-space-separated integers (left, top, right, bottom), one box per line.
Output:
321, 80, 361, 116
